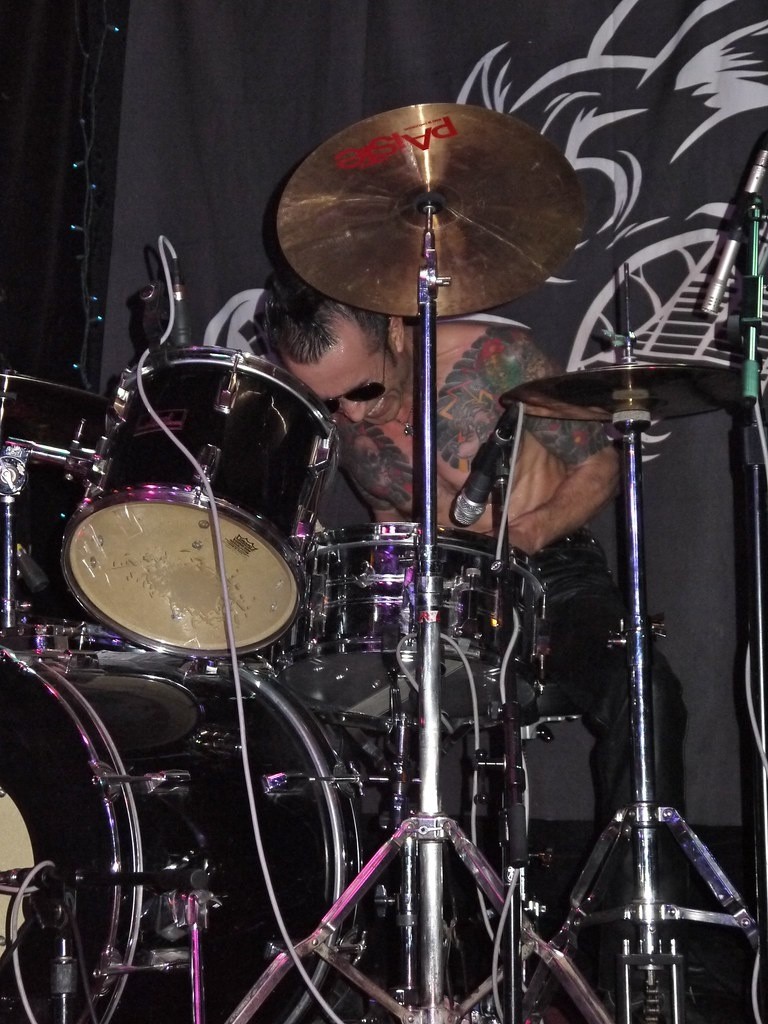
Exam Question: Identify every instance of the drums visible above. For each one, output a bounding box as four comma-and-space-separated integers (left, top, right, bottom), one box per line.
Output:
62, 345, 342, 658
271, 520, 548, 733
0, 642, 363, 1024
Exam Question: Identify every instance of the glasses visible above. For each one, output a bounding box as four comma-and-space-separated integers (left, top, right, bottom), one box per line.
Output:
323, 317, 392, 415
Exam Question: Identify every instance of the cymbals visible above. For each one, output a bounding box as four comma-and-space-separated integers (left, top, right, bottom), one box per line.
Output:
276, 103, 587, 317
499, 364, 743, 423
0, 370, 109, 401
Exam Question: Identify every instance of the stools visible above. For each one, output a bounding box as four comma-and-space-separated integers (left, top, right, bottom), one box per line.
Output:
504, 680, 589, 840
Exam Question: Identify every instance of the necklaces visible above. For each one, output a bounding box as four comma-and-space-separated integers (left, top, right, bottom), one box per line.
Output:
394, 407, 415, 435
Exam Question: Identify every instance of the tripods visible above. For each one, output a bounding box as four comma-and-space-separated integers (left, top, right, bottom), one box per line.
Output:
217, 206, 768, 1024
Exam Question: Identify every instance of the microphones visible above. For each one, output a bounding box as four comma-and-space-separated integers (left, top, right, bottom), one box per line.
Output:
702, 138, 768, 316
454, 403, 523, 526
172, 258, 191, 347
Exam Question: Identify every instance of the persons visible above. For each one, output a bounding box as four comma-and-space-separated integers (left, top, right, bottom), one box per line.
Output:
264, 272, 700, 990
